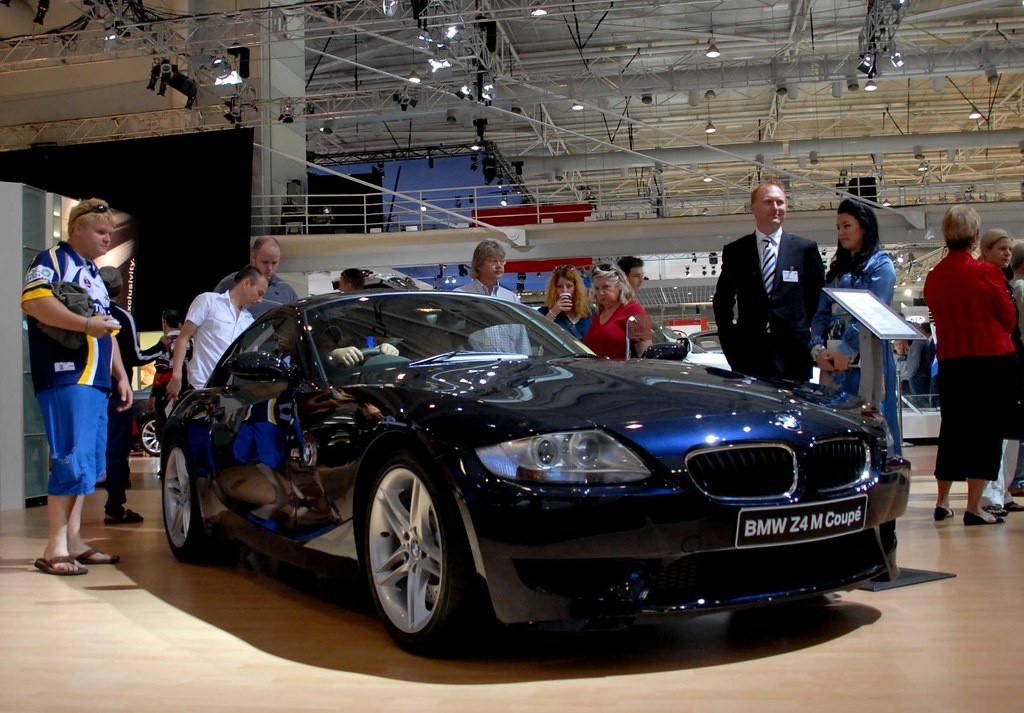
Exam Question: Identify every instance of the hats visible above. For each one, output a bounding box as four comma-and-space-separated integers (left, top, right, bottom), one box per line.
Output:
312, 318, 343, 347
100, 266, 124, 287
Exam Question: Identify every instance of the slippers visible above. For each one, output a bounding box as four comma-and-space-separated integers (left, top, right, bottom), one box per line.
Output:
34, 554, 89, 575
75, 548, 122, 564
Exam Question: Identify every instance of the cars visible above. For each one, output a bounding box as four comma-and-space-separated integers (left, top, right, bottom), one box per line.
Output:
130, 382, 162, 459
682, 331, 724, 355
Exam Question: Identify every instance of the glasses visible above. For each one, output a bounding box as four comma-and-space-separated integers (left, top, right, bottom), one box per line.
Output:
553, 264, 577, 273
591, 264, 615, 274
485, 257, 507, 267
74, 205, 107, 218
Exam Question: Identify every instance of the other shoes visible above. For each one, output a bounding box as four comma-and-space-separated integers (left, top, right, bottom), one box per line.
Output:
963, 511, 1006, 526
1010, 487, 1024, 496
103, 508, 145, 525
933, 507, 957, 521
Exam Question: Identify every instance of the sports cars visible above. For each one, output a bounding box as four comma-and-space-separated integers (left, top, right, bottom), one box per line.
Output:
157, 288, 914, 662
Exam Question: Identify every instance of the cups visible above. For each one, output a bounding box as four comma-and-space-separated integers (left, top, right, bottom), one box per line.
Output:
559, 292, 572, 307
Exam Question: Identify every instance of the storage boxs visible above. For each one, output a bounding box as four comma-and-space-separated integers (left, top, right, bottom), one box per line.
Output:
26, 0, 1024, 212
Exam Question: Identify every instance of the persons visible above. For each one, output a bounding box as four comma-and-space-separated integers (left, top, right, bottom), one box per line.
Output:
339, 268, 365, 292
20, 197, 133, 575
581, 262, 651, 361
146, 310, 193, 475
808, 198, 902, 582
1009, 243, 1024, 496
166, 265, 268, 407
617, 256, 644, 290
213, 237, 299, 321
538, 264, 598, 340
923, 204, 1016, 525
320, 308, 400, 370
100, 266, 167, 524
712, 180, 825, 382
977, 228, 1024, 516
892, 321, 940, 408
452, 240, 521, 304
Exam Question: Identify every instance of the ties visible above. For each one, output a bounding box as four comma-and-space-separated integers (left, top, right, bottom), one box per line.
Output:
760, 237, 777, 296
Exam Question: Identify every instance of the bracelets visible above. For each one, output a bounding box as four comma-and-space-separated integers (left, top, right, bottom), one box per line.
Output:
548, 309, 558, 320
85, 318, 91, 334
815, 346, 825, 355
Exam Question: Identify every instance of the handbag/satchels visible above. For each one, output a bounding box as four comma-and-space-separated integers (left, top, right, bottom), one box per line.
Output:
36, 281, 94, 352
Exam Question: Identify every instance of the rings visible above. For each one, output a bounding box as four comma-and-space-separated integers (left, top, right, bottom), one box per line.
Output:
109, 325, 114, 330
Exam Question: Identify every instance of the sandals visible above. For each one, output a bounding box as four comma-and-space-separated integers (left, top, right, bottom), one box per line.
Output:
983, 504, 1009, 517
1004, 501, 1024, 512
